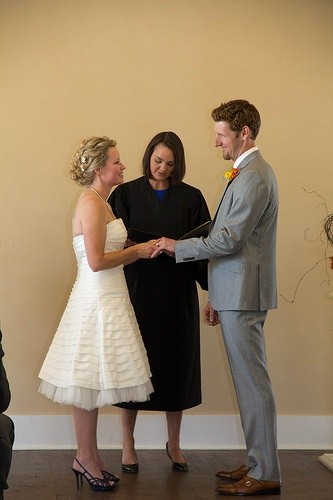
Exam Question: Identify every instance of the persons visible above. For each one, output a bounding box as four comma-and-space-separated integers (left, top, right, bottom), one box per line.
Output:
107, 131, 211, 473
150, 99, 282, 494
37, 135, 163, 490
0, 329, 15, 500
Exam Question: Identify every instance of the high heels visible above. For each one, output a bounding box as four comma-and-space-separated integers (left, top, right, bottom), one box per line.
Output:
165, 442, 189, 473
72, 458, 116, 491
80, 470, 120, 482
120, 451, 139, 474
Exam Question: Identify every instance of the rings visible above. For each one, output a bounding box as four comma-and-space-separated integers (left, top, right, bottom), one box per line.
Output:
160, 251, 163, 253
156, 243, 159, 246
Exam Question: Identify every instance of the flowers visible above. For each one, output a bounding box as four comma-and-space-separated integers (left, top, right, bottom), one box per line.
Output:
224, 166, 239, 179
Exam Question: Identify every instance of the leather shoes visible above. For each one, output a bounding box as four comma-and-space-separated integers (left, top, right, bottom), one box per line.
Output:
215, 464, 251, 480
215, 476, 281, 496
